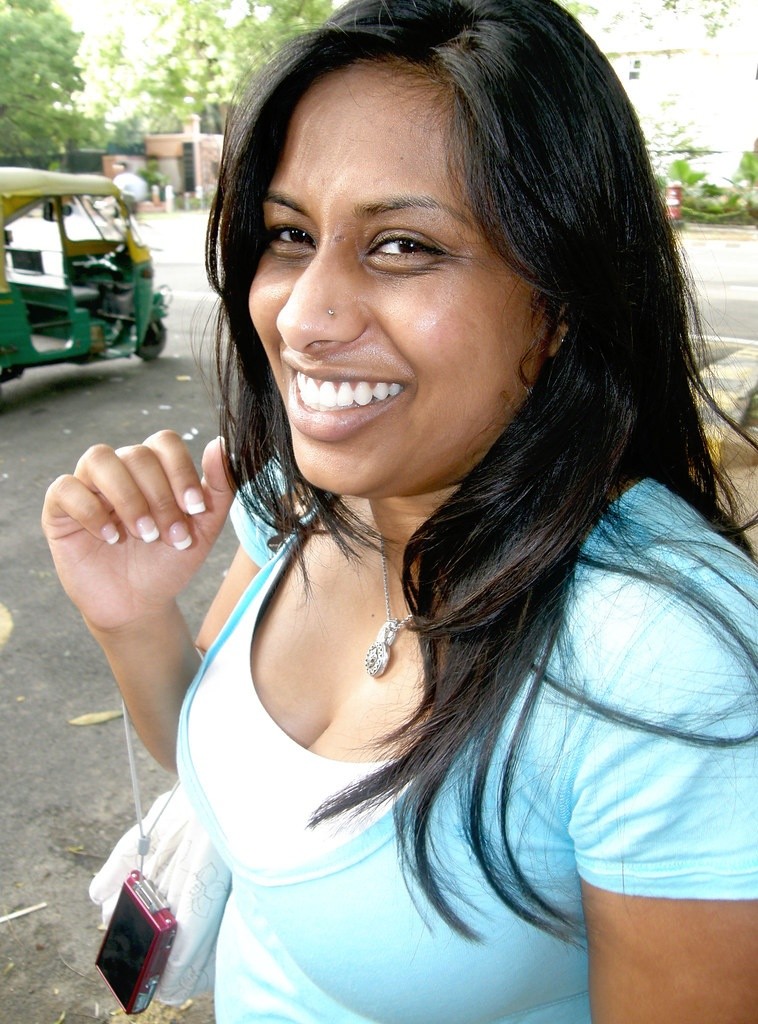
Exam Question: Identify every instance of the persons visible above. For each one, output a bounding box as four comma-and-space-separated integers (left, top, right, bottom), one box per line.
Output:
95, 162, 147, 218
41, 0, 758, 1024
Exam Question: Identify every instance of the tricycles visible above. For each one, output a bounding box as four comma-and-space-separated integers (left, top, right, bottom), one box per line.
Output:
0, 166, 170, 385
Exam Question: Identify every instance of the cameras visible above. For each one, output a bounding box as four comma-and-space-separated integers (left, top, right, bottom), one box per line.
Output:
94, 870, 177, 1016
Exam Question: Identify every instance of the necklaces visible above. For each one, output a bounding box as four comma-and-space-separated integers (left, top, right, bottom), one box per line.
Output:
366, 532, 412, 678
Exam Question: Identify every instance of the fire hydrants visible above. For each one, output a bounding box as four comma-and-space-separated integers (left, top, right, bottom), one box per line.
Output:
665, 179, 684, 219
